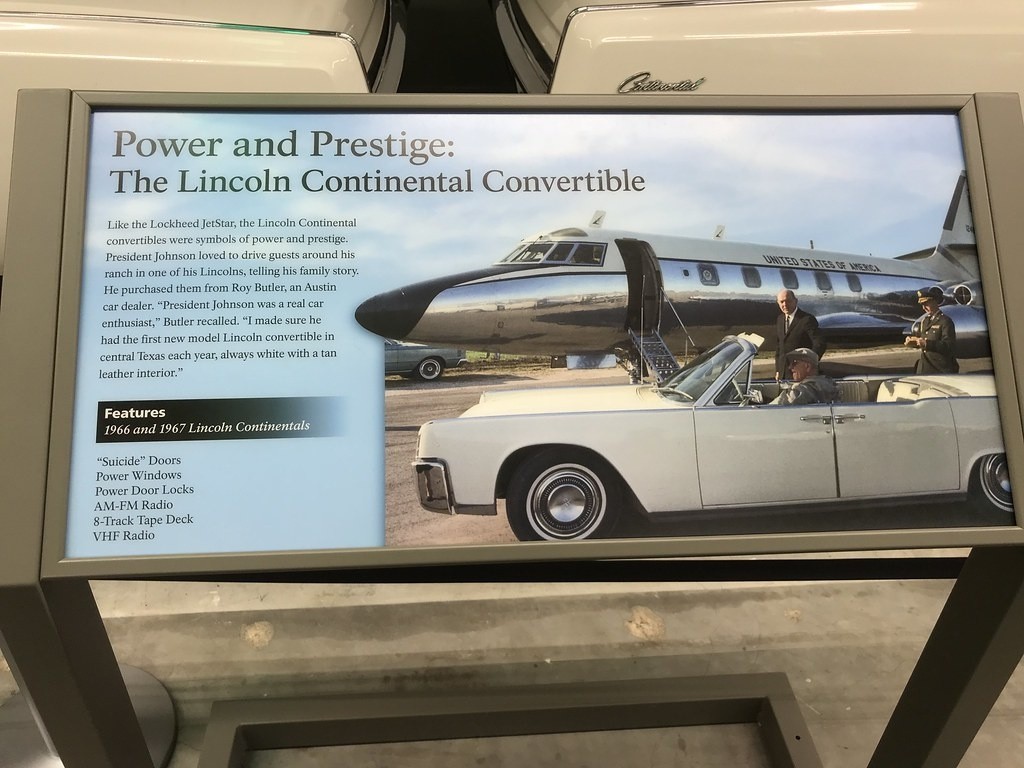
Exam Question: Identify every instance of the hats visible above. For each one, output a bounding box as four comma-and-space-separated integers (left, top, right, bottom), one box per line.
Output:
786, 347, 818, 369
917, 287, 943, 305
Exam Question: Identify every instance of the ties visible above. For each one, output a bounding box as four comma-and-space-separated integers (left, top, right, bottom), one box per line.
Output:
786, 316, 790, 333
927, 318, 932, 326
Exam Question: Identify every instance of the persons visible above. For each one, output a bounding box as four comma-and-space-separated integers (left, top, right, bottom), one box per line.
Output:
775, 289, 827, 381
904, 291, 960, 375
767, 348, 844, 406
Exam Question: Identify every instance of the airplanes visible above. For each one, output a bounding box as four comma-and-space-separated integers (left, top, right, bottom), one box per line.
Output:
355, 169, 992, 381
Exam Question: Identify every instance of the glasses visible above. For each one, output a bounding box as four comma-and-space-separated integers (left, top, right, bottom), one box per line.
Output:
792, 360, 801, 366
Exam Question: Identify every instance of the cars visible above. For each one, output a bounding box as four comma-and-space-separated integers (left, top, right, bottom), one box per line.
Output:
385, 337, 469, 382
410, 335, 1018, 543
